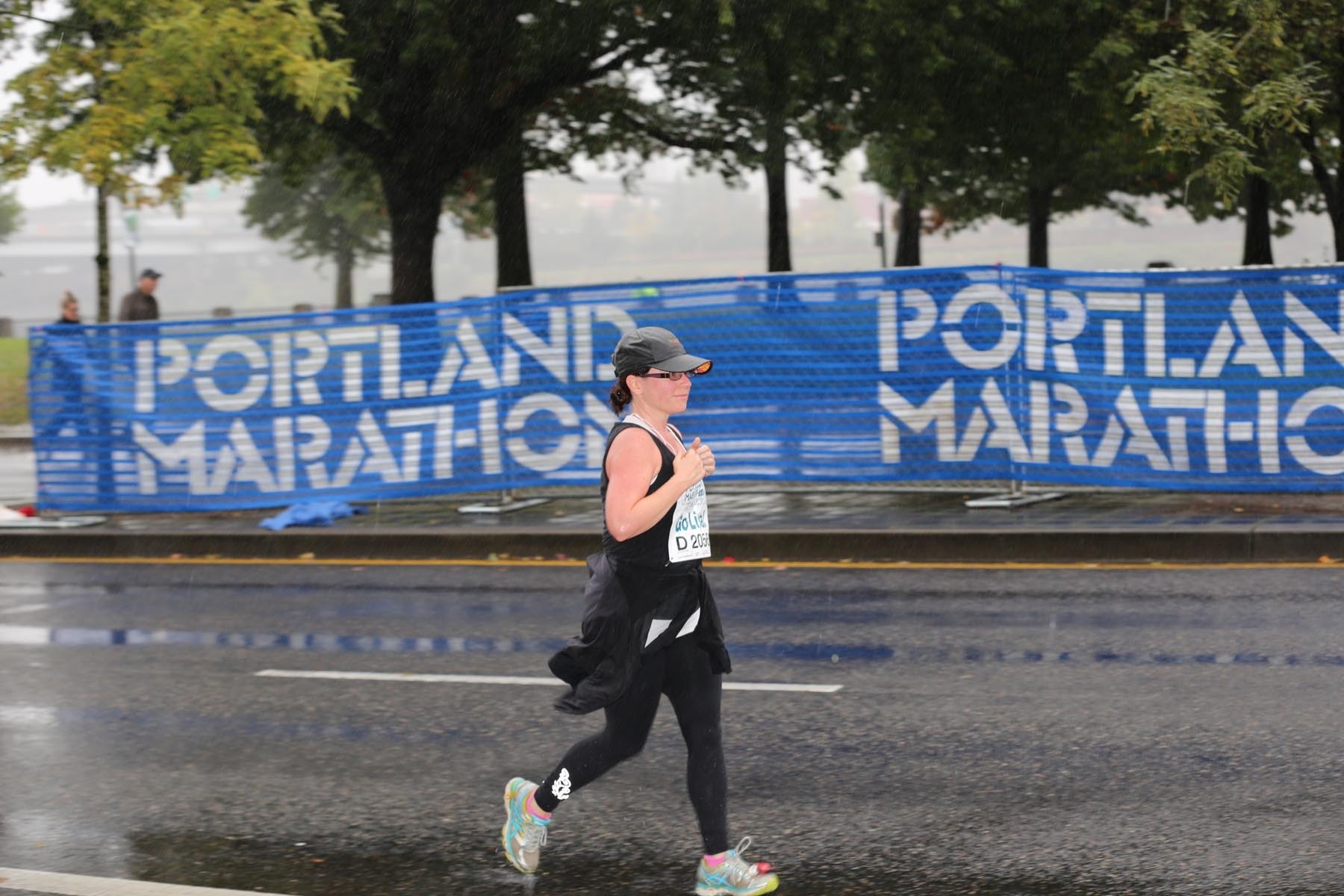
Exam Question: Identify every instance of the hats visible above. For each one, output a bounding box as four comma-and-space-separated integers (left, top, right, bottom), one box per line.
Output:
140, 267, 162, 279
613, 326, 713, 376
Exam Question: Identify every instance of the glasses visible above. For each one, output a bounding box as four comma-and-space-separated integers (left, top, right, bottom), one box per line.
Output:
640, 368, 696, 381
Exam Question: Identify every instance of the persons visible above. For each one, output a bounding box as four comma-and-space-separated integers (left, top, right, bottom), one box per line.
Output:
27, 269, 163, 447
500, 327, 779, 896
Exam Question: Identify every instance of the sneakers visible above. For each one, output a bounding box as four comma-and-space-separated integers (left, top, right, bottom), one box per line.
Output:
501, 776, 551, 876
695, 836, 781, 896
